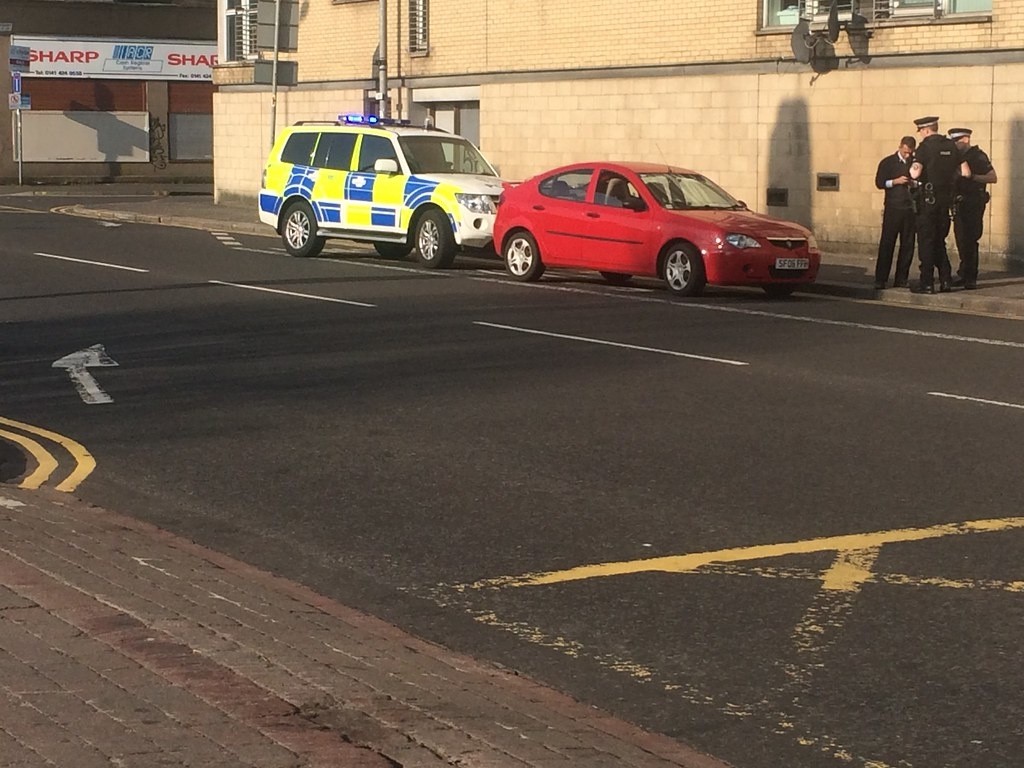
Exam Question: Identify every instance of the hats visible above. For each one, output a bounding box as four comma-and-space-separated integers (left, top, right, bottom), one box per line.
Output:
914, 116, 940, 132
948, 128, 972, 140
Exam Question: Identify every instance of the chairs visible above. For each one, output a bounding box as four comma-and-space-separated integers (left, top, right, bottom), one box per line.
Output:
549, 181, 570, 197
639, 182, 669, 208
604, 178, 623, 205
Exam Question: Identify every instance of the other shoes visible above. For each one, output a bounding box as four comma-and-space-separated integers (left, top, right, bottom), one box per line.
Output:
965, 278, 976, 289
955, 277, 964, 284
875, 281, 884, 288
895, 281, 911, 287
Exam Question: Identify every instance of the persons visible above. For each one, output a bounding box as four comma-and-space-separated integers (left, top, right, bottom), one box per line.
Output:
909, 117, 972, 296
873, 136, 916, 290
947, 129, 997, 291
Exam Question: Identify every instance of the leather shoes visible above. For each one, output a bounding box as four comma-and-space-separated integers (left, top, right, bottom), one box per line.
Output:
941, 281, 950, 291
911, 284, 934, 294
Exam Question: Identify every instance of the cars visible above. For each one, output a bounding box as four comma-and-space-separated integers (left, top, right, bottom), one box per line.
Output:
493, 161, 821, 298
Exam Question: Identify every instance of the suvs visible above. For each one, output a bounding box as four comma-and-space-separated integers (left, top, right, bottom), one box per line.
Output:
258, 113, 507, 270
